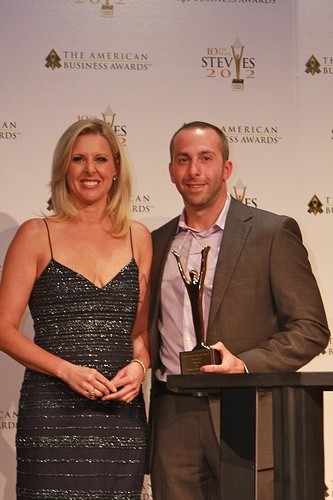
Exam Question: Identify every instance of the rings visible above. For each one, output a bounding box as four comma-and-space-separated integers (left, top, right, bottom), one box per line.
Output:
88, 387, 95, 398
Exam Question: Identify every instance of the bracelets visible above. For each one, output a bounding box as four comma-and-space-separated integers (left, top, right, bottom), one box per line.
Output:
129, 359, 146, 386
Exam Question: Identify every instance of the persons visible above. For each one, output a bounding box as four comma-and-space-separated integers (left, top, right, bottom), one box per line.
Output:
148, 122, 330, 500
0, 117, 154, 500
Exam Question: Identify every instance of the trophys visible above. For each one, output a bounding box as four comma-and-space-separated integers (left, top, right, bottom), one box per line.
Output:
169, 244, 221, 376
